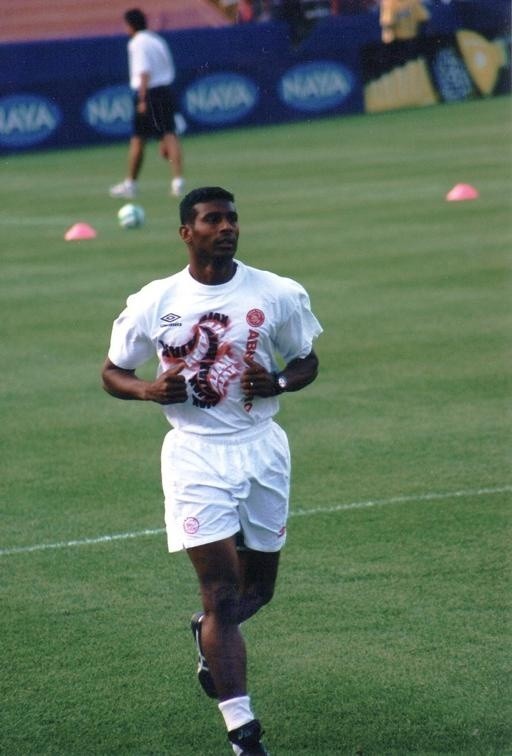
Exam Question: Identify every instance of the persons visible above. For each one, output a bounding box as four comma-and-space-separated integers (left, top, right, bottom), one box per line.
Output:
377, 0, 431, 73
107, 7, 188, 199
93, 182, 326, 752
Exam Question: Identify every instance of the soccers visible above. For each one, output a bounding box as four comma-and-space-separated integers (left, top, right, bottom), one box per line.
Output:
118, 203, 144, 228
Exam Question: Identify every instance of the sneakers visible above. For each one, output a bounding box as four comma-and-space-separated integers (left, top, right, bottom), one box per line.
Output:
172, 184, 184, 197
110, 183, 136, 200
191, 612, 218, 699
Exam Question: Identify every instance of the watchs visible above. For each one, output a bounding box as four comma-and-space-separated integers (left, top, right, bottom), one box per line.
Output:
273, 371, 286, 395
